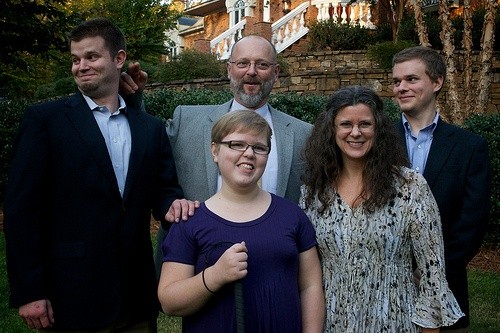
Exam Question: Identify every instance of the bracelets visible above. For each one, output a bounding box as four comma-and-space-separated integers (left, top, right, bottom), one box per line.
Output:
202, 268, 217, 295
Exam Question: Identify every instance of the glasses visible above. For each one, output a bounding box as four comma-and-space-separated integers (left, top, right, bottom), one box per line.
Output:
230, 60, 278, 70
213, 139, 270, 156
334, 122, 377, 130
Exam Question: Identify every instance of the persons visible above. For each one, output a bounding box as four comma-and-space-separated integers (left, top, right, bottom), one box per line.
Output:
298, 87, 465, 333
158, 110, 326, 333
390, 45, 492, 333
3, 18, 201, 333
119, 33, 316, 318
